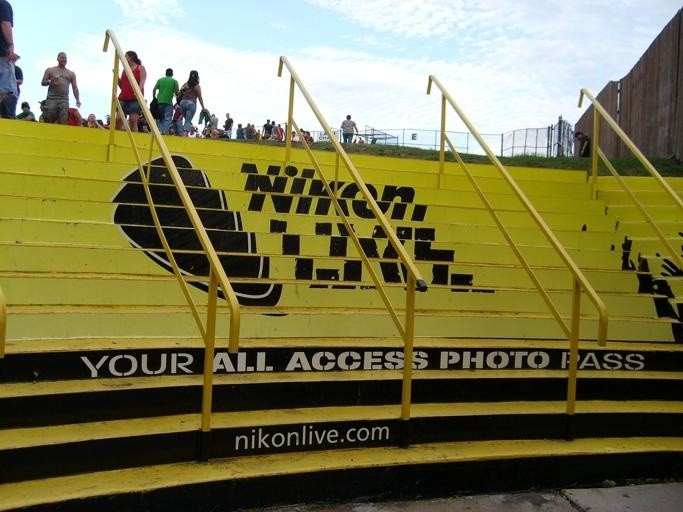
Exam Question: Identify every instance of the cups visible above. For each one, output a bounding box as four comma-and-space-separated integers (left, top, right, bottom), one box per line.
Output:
52, 74, 59, 85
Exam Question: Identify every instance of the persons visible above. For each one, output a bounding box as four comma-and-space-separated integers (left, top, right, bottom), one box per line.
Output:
177, 70, 205, 136
0, 0, 16, 118
40, 51, 81, 124
340, 114, 358, 142
14, 98, 313, 142
152, 68, 179, 134
13, 52, 24, 118
574, 131, 590, 157
111, 50, 146, 131
